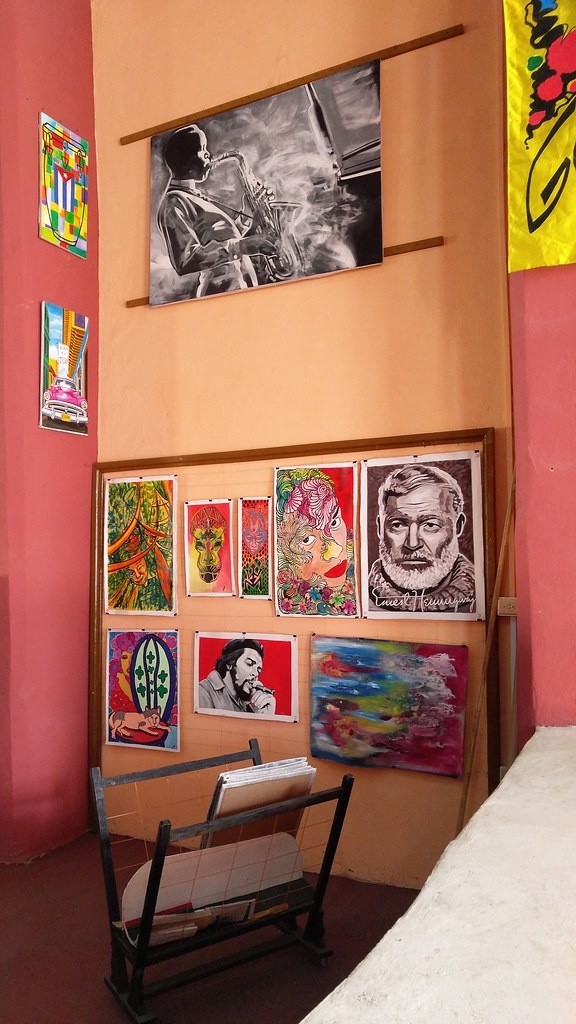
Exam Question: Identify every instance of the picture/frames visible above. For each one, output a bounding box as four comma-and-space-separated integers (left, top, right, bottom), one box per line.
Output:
192, 631, 299, 722
102, 475, 178, 616
184, 499, 236, 597
361, 450, 485, 621
238, 496, 272, 600
105, 628, 180, 752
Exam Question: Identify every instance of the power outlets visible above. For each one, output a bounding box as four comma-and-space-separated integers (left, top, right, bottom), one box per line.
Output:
496, 596, 517, 616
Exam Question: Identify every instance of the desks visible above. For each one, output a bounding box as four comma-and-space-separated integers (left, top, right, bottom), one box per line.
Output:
299, 723, 576, 1024
